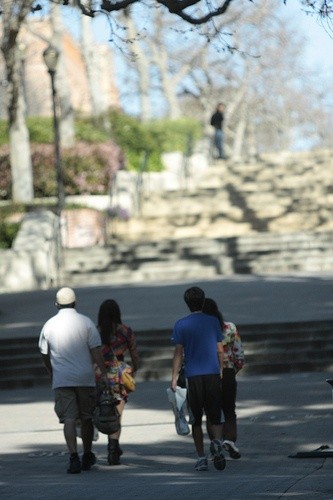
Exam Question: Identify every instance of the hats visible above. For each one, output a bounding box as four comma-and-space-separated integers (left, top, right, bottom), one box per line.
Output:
56, 287, 76, 305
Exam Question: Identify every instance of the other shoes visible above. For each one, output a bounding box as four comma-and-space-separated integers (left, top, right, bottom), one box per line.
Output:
82, 452, 96, 471
67, 452, 81, 473
223, 440, 241, 459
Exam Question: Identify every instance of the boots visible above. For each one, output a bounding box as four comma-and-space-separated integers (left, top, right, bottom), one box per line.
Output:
107, 439, 122, 465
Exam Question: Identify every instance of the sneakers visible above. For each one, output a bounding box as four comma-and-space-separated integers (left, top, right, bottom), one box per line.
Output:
192, 456, 208, 471
210, 439, 226, 471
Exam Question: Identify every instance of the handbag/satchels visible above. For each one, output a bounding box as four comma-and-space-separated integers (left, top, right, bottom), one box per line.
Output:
176, 367, 186, 389
118, 361, 136, 392
167, 386, 190, 435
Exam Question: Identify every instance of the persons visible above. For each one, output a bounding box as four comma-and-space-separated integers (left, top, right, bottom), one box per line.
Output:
210, 102, 228, 159
170, 286, 245, 472
38, 287, 106, 475
91, 297, 139, 466
